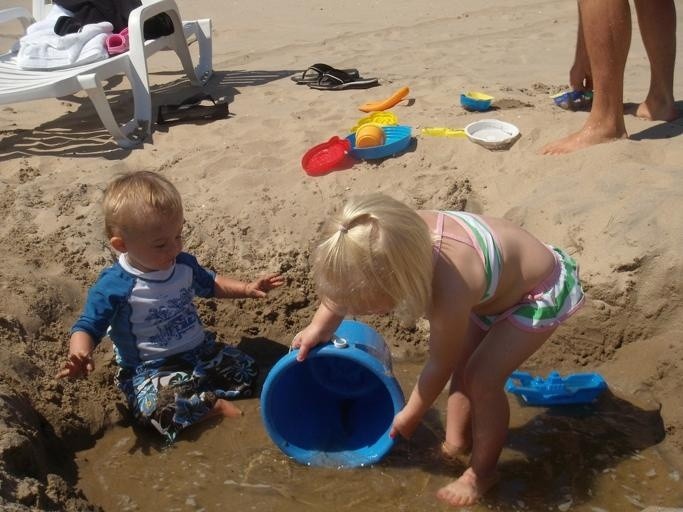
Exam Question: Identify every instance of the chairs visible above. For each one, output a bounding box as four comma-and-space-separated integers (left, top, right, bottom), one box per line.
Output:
2, 0, 214, 150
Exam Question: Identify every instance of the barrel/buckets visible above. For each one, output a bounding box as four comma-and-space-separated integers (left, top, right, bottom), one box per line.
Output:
260, 320, 406, 468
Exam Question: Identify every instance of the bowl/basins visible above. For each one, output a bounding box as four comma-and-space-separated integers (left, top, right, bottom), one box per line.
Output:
460, 95, 491, 111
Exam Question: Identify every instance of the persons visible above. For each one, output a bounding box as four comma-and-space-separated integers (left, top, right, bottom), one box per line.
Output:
538, 1, 678, 153
291, 190, 581, 505
57, 175, 286, 444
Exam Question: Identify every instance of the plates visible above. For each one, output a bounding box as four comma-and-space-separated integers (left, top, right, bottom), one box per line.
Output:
464, 118, 519, 145
346, 126, 412, 159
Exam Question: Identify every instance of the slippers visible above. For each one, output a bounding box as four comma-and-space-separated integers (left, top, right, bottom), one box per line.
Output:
107, 34, 126, 53
159, 92, 228, 123
120, 27, 129, 49
311, 69, 378, 90
291, 63, 359, 83
